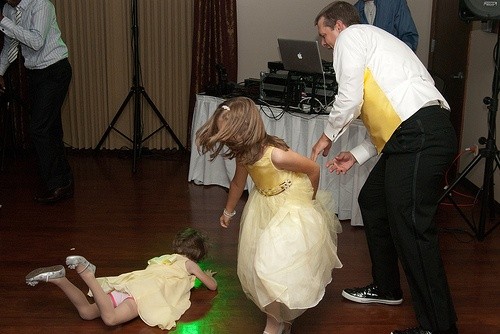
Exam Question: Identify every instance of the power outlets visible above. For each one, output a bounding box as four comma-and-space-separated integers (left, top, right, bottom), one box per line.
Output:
472, 144, 479, 156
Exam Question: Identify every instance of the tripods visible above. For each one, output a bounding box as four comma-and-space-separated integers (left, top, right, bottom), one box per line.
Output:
439, 27, 500, 243
91, 0, 190, 174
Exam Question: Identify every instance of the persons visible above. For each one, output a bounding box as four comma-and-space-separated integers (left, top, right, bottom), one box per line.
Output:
26, 228, 218, 330
0, 0, 74, 204
311, 1, 458, 334
195, 97, 343, 334
354, 0, 419, 53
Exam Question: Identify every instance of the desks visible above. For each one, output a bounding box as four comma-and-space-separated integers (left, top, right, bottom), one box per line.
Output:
188, 92, 383, 227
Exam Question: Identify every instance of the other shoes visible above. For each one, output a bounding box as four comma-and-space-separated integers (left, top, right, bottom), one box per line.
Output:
65, 256, 96, 275
390, 324, 459, 334
342, 283, 404, 305
263, 321, 292, 334
46, 178, 75, 202
25, 265, 65, 287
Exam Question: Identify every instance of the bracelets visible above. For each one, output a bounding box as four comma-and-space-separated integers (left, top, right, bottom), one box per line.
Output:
223, 209, 236, 217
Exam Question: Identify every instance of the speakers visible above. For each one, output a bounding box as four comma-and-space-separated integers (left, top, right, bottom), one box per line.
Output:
458, 0, 500, 24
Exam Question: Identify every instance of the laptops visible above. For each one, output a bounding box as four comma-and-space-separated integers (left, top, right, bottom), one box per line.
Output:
278, 38, 336, 75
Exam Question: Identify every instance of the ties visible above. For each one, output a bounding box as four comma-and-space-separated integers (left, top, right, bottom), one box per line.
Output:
8, 7, 23, 63
